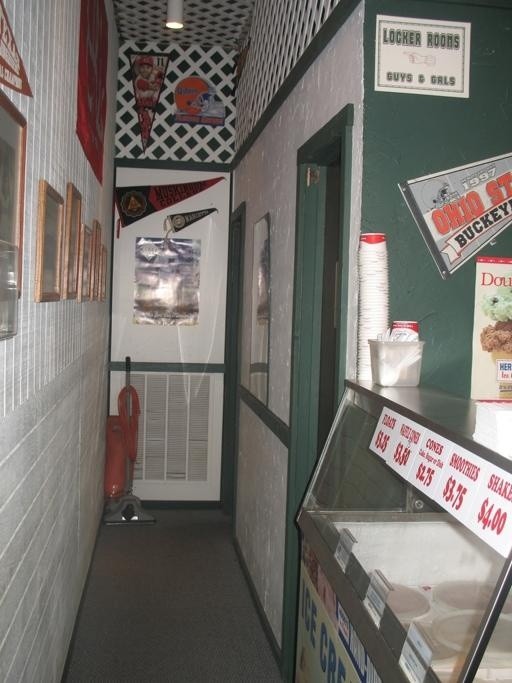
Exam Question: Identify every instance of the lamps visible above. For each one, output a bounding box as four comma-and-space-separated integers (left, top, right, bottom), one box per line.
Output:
167, 0, 186, 29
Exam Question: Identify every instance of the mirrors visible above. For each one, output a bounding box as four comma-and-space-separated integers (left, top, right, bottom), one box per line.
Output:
248, 210, 270, 407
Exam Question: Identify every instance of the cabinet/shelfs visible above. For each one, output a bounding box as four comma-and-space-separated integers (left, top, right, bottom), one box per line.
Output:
296, 382, 512, 682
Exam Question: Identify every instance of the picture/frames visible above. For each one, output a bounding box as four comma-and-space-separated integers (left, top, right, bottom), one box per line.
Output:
0, 89, 107, 338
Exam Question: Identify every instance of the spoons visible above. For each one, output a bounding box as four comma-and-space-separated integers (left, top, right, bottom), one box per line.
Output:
382, 350, 422, 385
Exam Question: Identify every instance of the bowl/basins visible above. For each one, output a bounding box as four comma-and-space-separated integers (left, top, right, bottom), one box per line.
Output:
392, 320, 419, 341
358, 232, 388, 385
